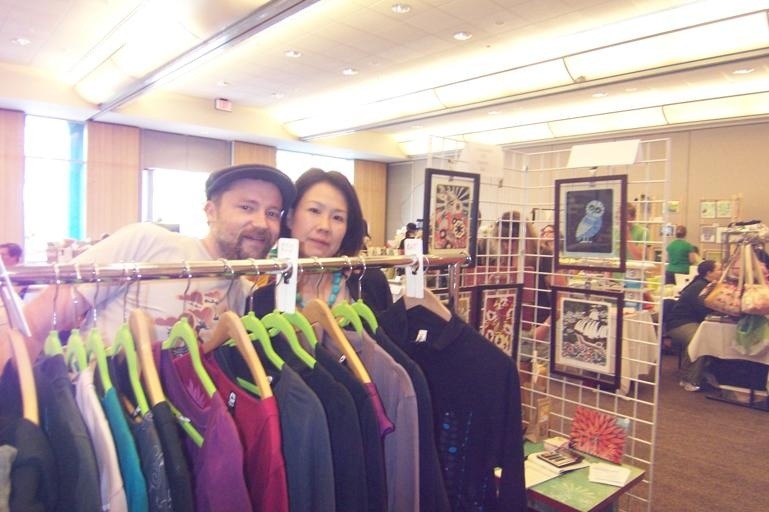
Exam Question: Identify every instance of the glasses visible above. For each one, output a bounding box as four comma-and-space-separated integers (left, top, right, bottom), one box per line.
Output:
540, 230, 555, 237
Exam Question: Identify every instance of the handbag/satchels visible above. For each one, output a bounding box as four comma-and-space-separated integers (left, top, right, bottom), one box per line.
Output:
702, 279, 769, 357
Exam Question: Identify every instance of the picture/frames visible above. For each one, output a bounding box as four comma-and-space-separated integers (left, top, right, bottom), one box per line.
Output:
548, 285, 624, 388
553, 174, 627, 273
432, 283, 524, 366
422, 167, 480, 269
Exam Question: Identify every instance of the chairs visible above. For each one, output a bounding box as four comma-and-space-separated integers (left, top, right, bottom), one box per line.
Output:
661, 297, 685, 373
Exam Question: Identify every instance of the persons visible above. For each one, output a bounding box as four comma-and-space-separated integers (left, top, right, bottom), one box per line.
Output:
496, 211, 563, 345
1, 242, 28, 298
665, 225, 696, 285
666, 260, 723, 391
242, 167, 393, 324
398, 223, 419, 255
1, 164, 297, 373
625, 203, 652, 261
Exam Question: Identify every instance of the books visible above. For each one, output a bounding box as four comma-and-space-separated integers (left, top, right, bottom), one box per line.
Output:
524, 446, 630, 489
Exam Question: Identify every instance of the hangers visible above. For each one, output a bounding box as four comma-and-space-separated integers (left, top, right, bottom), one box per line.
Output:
160, 256, 223, 447
301, 256, 377, 391
76, 253, 129, 425
340, 255, 426, 377
63, 265, 106, 421
377, 256, 511, 368
117, 258, 167, 405
2, 256, 44, 431
329, 255, 409, 380
200, 255, 277, 402
103, 259, 149, 422
40, 255, 80, 422
268, 254, 355, 385
230, 256, 338, 390
221, 256, 314, 392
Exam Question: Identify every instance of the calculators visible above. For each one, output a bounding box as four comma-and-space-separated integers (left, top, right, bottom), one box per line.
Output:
536, 446, 585, 468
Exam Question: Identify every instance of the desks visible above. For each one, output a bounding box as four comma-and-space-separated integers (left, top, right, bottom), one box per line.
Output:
686, 313, 769, 411
494, 435, 647, 512
548, 308, 658, 397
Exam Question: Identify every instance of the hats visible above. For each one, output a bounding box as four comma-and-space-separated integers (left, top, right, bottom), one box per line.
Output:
205, 163, 297, 209
406, 222, 420, 231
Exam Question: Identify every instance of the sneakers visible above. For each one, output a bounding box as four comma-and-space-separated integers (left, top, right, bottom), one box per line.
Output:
679, 380, 702, 392
